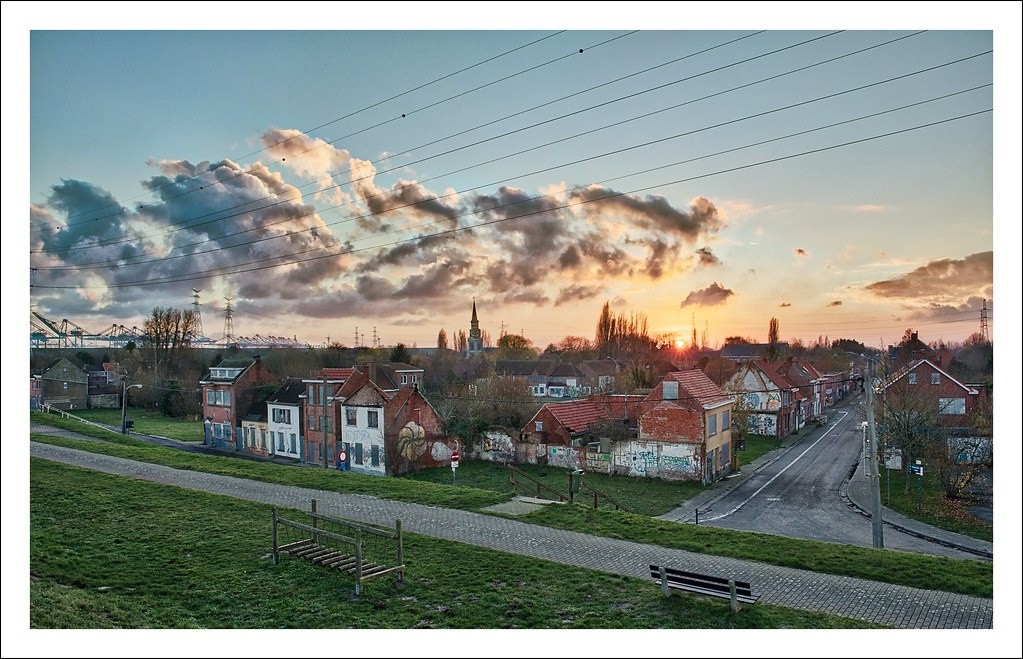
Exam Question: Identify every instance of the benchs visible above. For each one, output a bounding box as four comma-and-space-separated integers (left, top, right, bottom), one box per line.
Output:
649, 564, 762, 613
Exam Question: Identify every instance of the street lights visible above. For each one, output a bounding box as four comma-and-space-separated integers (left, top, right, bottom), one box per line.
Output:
122, 381, 143, 434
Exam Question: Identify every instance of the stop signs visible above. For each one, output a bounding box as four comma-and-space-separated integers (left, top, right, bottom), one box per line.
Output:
451, 451, 459, 462
